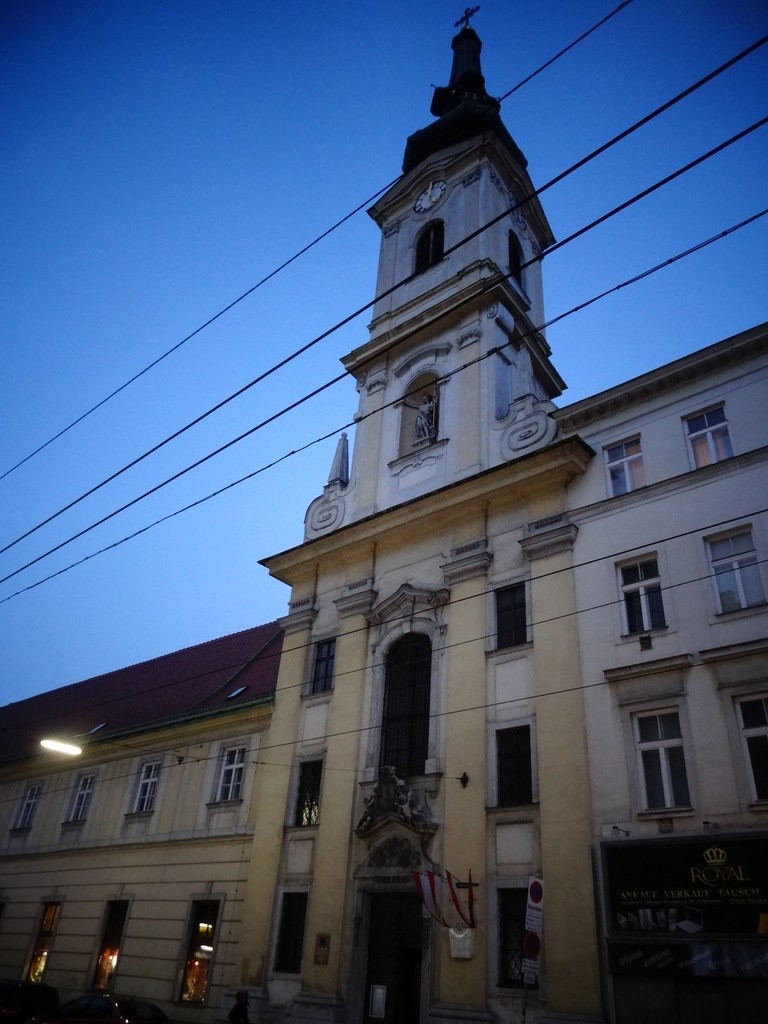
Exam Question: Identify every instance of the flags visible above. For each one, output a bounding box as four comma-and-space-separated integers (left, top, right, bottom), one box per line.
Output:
447, 870, 471, 928
425, 870, 449, 928
410, 870, 446, 928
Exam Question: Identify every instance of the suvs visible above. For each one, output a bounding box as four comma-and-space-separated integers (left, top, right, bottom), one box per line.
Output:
0, 978, 60, 1024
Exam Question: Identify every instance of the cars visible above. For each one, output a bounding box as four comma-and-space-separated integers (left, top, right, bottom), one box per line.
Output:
44, 993, 170, 1024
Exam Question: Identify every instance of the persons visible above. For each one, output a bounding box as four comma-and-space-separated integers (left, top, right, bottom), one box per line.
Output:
228, 990, 251, 1024
